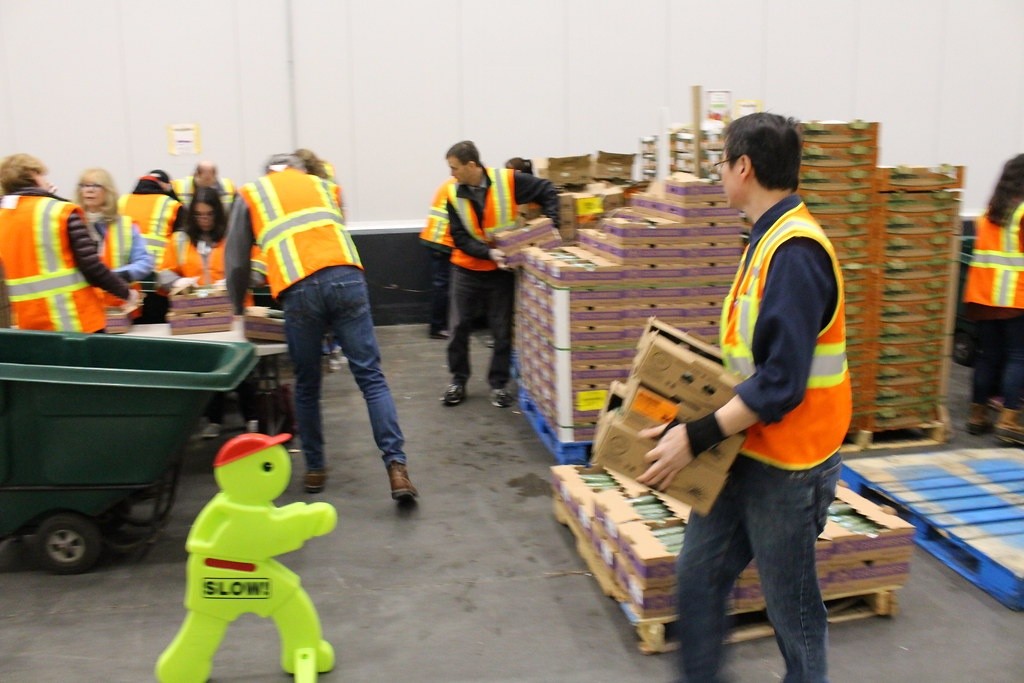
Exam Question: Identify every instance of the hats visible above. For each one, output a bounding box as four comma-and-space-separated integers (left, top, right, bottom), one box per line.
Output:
151, 170, 170, 183
267, 154, 305, 170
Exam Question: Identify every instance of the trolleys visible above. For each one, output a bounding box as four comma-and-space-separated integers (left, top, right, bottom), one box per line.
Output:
0, 326, 262, 574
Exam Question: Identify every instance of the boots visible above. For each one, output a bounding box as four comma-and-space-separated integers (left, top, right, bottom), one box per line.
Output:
303, 469, 330, 493
387, 465, 420, 500
965, 402, 993, 435
994, 408, 1023, 447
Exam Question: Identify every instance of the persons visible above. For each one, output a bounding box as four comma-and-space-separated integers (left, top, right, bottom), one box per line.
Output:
118, 170, 187, 324
292, 149, 347, 370
638, 113, 853, 683
171, 160, 238, 218
223, 154, 419, 501
154, 186, 255, 306
75, 165, 153, 323
438, 140, 561, 408
0, 152, 140, 334
961, 153, 1024, 444
505, 158, 540, 221
419, 175, 457, 338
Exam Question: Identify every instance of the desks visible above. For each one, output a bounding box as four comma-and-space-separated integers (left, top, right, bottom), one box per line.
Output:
119, 315, 289, 440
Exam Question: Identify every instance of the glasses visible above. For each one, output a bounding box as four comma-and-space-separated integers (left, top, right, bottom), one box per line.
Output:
715, 158, 735, 173
78, 183, 104, 190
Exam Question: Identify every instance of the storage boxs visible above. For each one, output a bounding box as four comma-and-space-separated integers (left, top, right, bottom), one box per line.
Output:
492, 118, 966, 620
165, 288, 233, 336
106, 309, 130, 333
246, 306, 286, 342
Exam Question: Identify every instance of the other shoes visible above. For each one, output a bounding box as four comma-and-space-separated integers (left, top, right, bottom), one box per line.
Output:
430, 328, 448, 338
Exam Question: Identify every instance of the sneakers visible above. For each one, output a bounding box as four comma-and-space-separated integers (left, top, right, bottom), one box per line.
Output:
488, 389, 512, 408
444, 385, 467, 404
